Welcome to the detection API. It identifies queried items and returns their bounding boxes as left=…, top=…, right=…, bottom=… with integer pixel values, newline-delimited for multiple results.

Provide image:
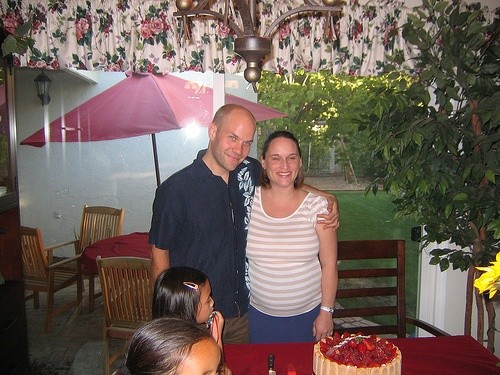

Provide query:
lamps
left=33, top=71, right=51, bottom=106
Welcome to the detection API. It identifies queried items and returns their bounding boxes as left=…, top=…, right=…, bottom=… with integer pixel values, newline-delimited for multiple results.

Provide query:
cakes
left=313, top=332, right=402, bottom=375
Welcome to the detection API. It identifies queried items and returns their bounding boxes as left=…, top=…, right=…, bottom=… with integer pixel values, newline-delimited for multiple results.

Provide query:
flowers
left=473, top=252, right=500, bottom=299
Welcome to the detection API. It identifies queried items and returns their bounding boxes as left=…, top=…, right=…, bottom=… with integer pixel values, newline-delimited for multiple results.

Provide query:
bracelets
left=320, top=305, right=333, bottom=313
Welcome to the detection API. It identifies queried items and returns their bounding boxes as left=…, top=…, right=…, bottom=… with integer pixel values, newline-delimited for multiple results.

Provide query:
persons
left=243, top=131, right=338, bottom=345
left=149, top=103, right=340, bottom=344
left=123, top=266, right=232, bottom=375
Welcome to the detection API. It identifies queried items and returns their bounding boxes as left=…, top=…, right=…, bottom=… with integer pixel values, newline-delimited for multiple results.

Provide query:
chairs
left=19, top=205, right=125, bottom=334
left=317, top=239, right=451, bottom=338
left=96, top=255, right=154, bottom=375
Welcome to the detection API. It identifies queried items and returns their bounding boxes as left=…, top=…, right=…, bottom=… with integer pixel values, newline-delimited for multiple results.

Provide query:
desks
left=79, top=232, right=152, bottom=313
left=222, top=336, right=500, bottom=375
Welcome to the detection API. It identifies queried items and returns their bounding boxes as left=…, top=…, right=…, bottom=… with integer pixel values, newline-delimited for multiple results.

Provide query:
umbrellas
left=20, top=63, right=289, bottom=186
